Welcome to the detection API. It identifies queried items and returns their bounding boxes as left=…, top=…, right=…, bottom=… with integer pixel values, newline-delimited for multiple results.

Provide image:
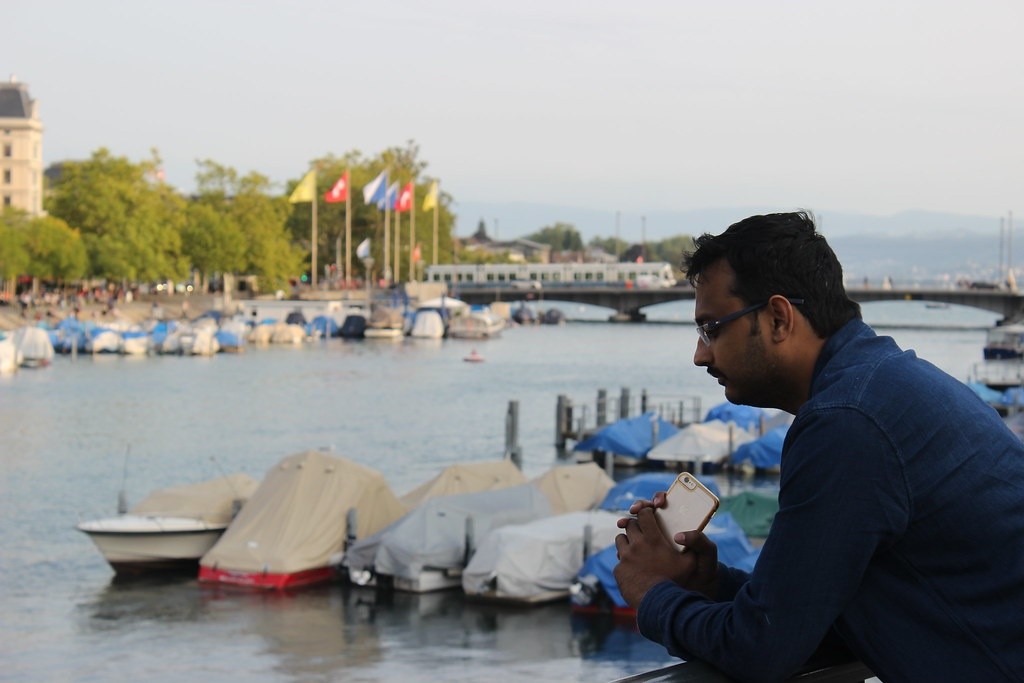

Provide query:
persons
left=615, top=212, right=1024, bottom=683
left=18, top=282, right=125, bottom=319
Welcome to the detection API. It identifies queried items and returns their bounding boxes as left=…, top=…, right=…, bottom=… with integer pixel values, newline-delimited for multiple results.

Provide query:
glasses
left=693, top=295, right=806, bottom=348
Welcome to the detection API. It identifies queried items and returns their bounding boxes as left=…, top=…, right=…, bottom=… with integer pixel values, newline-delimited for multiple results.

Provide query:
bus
left=427, top=262, right=679, bottom=288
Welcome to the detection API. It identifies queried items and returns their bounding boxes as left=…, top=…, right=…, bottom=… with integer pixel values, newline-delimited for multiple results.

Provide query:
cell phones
left=654, top=471, right=720, bottom=555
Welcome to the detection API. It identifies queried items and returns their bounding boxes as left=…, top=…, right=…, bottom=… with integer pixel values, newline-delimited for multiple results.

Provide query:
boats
left=339, top=485, right=552, bottom=596
left=1, top=301, right=568, bottom=376
left=458, top=503, right=630, bottom=604
left=198, top=449, right=403, bottom=590
left=73, top=471, right=257, bottom=577
left=731, top=428, right=786, bottom=477
left=568, top=523, right=755, bottom=632
left=647, top=420, right=760, bottom=476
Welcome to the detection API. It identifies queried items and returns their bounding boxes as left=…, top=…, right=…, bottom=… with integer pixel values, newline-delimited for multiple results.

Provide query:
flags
left=377, top=181, right=396, bottom=211
left=362, top=169, right=386, bottom=204
left=355, top=237, right=371, bottom=258
left=287, top=168, right=317, bottom=204
left=422, top=182, right=435, bottom=211
left=394, top=183, right=412, bottom=210
left=323, top=169, right=347, bottom=203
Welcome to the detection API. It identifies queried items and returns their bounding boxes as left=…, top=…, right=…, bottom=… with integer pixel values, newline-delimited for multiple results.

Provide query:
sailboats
left=575, top=409, right=682, bottom=467
